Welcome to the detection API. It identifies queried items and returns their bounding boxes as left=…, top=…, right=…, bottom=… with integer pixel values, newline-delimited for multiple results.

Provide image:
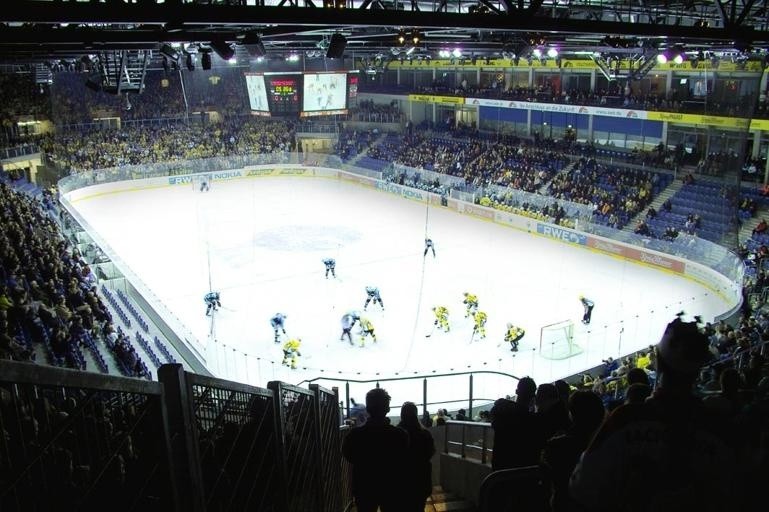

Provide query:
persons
left=204, top=291, right=222, bottom=316
left=433, top=305, right=450, bottom=332
left=341, top=312, right=376, bottom=347
left=424, top=238, right=435, bottom=256
left=324, top=258, right=336, bottom=278
left=0, top=226, right=117, bottom=361
left=2, top=323, right=769, bottom=511
left=271, top=313, right=286, bottom=342
left=736, top=219, right=768, bottom=328
left=364, top=286, right=384, bottom=311
left=463, top=293, right=487, bottom=338
left=579, top=295, right=593, bottom=323
left=1, top=50, right=769, bottom=227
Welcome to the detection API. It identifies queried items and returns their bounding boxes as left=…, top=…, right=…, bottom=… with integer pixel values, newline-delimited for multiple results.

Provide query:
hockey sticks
left=426, top=325, right=437, bottom=337
